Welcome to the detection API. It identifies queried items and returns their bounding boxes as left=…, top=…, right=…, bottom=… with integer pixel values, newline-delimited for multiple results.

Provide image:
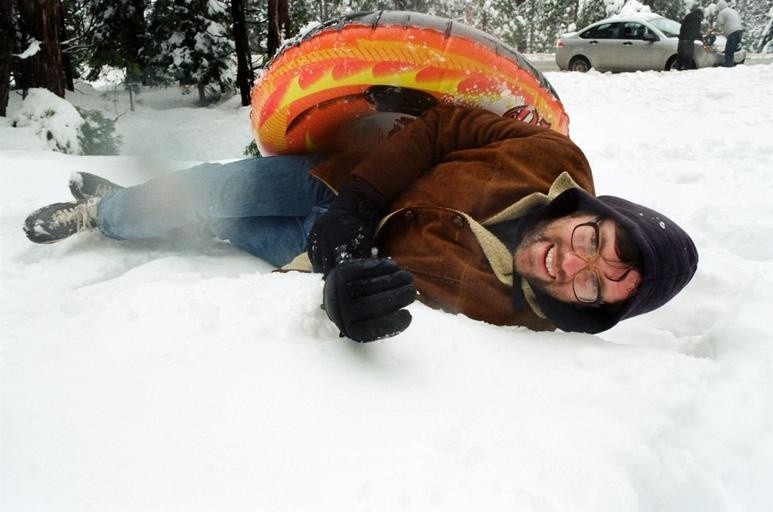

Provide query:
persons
left=709, top=0, right=743, bottom=67
left=22, top=102, right=699, bottom=341
left=673, top=7, right=704, bottom=71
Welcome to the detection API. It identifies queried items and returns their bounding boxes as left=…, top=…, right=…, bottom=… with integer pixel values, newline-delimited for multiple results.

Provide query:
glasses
left=571, top=215, right=604, bottom=309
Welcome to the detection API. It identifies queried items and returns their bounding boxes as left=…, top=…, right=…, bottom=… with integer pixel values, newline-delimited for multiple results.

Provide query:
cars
left=555, top=11, right=745, bottom=74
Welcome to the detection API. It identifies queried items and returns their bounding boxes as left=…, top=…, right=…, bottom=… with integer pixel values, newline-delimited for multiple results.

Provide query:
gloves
left=321, top=258, right=416, bottom=341
left=305, top=192, right=377, bottom=272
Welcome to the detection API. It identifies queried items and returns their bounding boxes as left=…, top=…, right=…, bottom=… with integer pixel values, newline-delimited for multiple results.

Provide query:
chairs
left=636, top=25, right=645, bottom=39
left=624, top=25, right=636, bottom=39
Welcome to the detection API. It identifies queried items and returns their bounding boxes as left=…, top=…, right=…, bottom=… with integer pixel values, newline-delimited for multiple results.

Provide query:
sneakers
left=70, top=172, right=124, bottom=201
left=24, top=201, right=97, bottom=244
